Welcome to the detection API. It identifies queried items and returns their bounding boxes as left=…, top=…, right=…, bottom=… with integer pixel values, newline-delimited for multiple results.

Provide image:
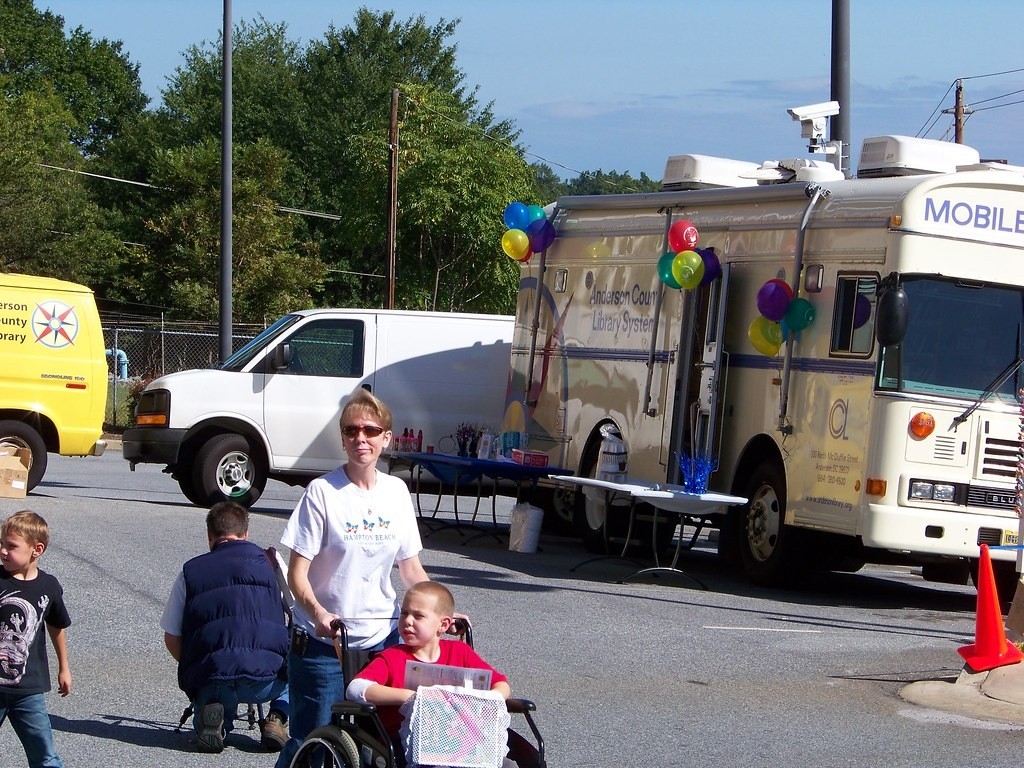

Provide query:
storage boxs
left=510, top=449, right=548, bottom=469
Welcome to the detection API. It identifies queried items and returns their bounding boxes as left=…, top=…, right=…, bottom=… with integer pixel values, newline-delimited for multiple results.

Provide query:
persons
left=0, top=509, right=72, bottom=768
left=343, top=582, right=531, bottom=768
left=275, top=388, right=471, bottom=768
left=160, top=502, right=295, bottom=750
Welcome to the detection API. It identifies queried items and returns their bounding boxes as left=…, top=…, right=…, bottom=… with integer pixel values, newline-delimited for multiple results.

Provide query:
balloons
left=527, top=205, right=546, bottom=223
left=748, top=279, right=814, bottom=357
left=580, top=242, right=613, bottom=270
left=656, top=251, right=684, bottom=289
left=699, top=249, right=720, bottom=287
left=504, top=202, right=530, bottom=231
left=672, top=251, right=705, bottom=290
left=516, top=247, right=532, bottom=262
left=668, top=219, right=699, bottom=253
left=528, top=219, right=556, bottom=254
left=853, top=293, right=872, bottom=331
left=502, top=229, right=529, bottom=260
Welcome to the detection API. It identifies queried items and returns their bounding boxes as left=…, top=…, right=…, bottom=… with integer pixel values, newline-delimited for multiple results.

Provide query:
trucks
left=0, top=272, right=108, bottom=493
left=500, top=101, right=1024, bottom=584
left=122, top=309, right=516, bottom=509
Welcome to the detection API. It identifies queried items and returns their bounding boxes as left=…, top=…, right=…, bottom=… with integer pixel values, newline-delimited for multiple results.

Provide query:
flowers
left=455, top=421, right=486, bottom=440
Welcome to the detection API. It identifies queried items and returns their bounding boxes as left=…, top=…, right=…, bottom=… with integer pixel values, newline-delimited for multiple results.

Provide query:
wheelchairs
left=289, top=618, right=548, bottom=768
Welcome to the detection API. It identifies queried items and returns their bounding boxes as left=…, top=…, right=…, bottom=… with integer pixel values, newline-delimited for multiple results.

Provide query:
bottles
left=400, top=428, right=424, bottom=452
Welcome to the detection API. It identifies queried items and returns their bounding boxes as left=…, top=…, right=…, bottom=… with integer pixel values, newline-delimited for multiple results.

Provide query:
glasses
left=342, top=425, right=385, bottom=436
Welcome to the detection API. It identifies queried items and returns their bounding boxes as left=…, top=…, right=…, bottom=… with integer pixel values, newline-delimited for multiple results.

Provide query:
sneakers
left=196, top=699, right=224, bottom=753
left=260, top=715, right=291, bottom=749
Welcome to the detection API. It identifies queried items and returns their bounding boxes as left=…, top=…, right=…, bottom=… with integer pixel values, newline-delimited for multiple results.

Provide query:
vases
left=457, top=438, right=478, bottom=459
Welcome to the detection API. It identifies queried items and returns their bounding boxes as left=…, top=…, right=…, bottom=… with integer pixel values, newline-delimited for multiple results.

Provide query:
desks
left=378, top=449, right=575, bottom=548
left=547, top=474, right=750, bottom=591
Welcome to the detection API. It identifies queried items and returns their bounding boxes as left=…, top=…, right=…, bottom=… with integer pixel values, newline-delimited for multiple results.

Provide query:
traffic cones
left=957, top=544, right=1023, bottom=672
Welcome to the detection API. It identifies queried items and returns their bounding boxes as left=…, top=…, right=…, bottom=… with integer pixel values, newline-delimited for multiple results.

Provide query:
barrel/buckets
left=509, top=508, right=544, bottom=553
